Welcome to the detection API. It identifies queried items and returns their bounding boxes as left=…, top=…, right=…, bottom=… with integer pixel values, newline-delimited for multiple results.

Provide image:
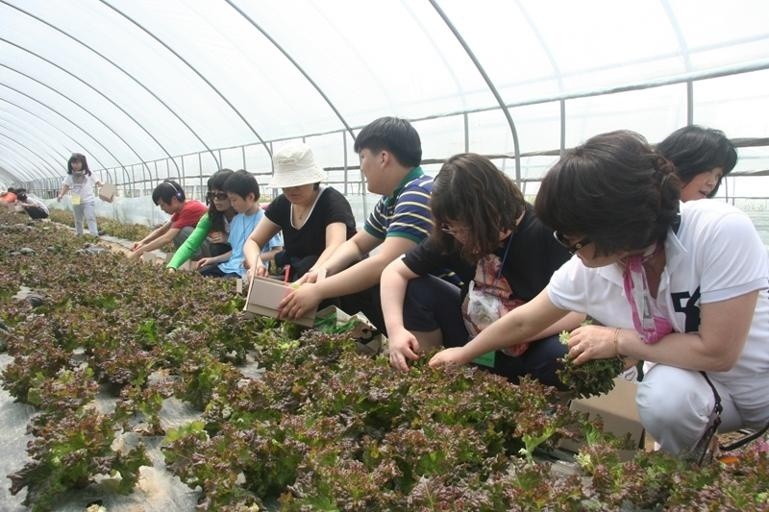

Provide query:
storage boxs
left=99, top=183, right=115, bottom=203
left=565, top=377, right=655, bottom=453
left=243, top=254, right=328, bottom=329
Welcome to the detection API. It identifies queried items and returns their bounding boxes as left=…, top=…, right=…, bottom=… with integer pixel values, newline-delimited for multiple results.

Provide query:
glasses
left=206, top=191, right=228, bottom=200
left=551, top=227, right=593, bottom=256
left=438, top=222, right=475, bottom=237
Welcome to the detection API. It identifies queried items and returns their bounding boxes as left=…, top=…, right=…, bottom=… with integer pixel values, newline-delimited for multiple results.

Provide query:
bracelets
left=613, top=326, right=622, bottom=355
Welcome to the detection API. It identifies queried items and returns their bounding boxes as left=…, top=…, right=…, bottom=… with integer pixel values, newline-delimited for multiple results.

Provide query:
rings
left=574, top=345, right=579, bottom=354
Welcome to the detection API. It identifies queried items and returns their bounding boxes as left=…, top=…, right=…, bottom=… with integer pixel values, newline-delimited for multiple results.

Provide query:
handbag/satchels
left=70, top=194, right=82, bottom=206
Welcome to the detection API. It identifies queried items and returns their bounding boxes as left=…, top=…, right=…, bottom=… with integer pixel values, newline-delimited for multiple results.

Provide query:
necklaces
left=295, top=202, right=310, bottom=220
left=242, top=208, right=259, bottom=242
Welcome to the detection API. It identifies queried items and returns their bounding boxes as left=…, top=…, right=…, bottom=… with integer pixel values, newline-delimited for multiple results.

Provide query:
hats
left=265, top=142, right=329, bottom=189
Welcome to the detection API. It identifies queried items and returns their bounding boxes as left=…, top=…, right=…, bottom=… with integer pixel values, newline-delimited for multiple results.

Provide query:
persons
left=279, top=117, right=436, bottom=338
left=0, top=187, right=49, bottom=222
left=242, top=142, right=357, bottom=285
left=427, top=130, right=769, bottom=455
left=378, top=153, right=576, bottom=390
left=56, top=154, right=104, bottom=237
left=166, top=168, right=237, bottom=276
left=195, top=169, right=284, bottom=278
left=655, top=124, right=738, bottom=204
left=129, top=178, right=210, bottom=262
